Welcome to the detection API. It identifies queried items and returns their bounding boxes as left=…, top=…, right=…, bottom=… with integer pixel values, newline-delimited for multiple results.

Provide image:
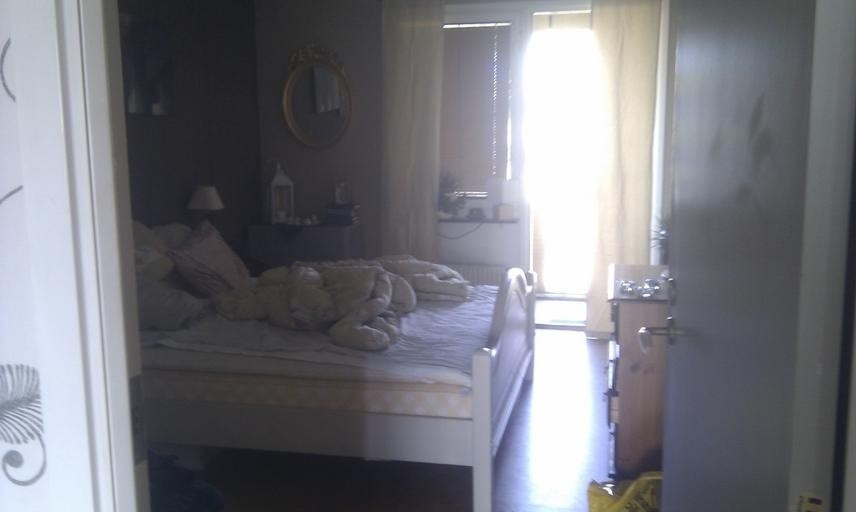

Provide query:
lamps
left=186, top=186, right=225, bottom=218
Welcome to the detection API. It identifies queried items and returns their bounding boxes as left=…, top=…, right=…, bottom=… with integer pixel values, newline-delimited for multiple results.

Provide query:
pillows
left=133, top=220, right=250, bottom=329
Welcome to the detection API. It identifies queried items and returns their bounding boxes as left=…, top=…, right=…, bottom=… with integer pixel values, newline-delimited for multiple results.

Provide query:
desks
left=248, top=222, right=364, bottom=268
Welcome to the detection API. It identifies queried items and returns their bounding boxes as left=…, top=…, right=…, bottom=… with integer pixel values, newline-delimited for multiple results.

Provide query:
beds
left=140, top=266, right=535, bottom=512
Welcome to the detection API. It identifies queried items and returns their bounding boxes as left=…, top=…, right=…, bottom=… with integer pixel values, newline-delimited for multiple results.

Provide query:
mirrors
left=282, top=43, right=353, bottom=150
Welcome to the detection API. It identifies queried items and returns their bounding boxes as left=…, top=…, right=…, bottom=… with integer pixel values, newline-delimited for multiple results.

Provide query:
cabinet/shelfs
left=606, top=265, right=668, bottom=479
